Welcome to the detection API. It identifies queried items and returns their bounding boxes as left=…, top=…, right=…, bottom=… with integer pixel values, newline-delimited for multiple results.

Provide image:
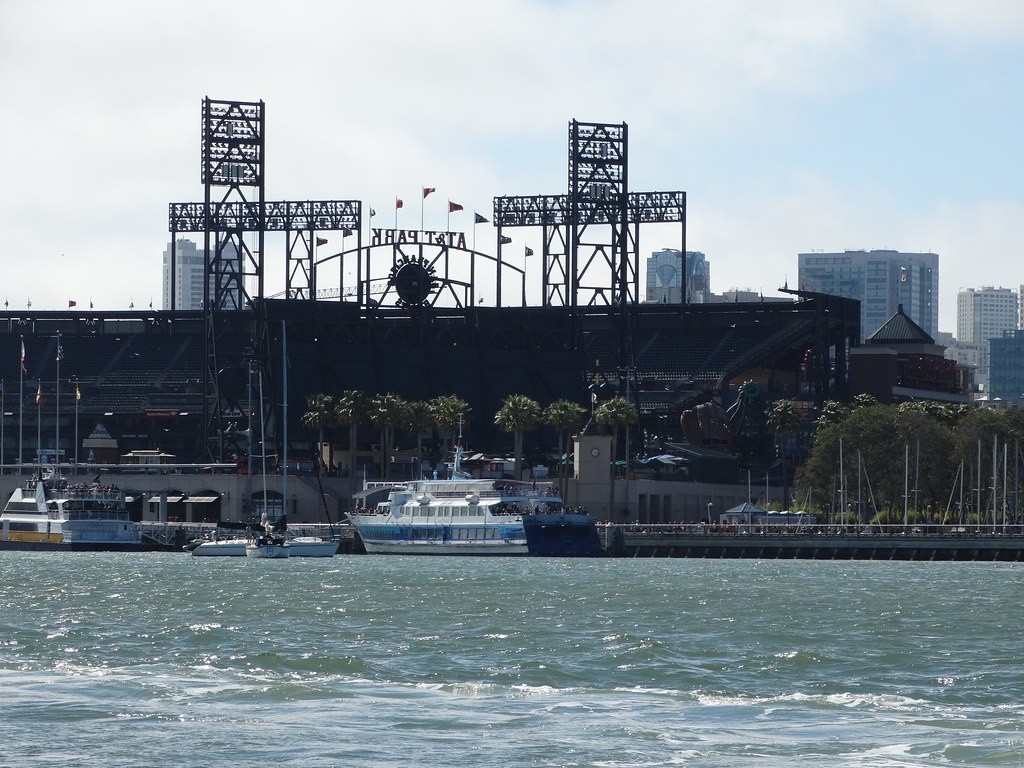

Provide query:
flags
left=58, top=345, right=65, bottom=360
left=424, top=188, right=435, bottom=198
left=525, top=247, right=533, bottom=256
left=450, top=202, right=463, bottom=212
left=5, top=300, right=8, bottom=307
left=397, top=199, right=402, bottom=208
left=317, top=237, right=328, bottom=246
left=500, top=235, right=511, bottom=244
left=21, top=340, right=25, bottom=363
left=591, top=393, right=598, bottom=403
left=69, top=301, right=76, bottom=307
left=344, top=228, right=352, bottom=237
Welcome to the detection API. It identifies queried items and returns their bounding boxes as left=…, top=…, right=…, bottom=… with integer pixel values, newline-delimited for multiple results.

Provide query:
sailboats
left=837, top=434, right=1024, bottom=534
left=182, top=320, right=341, bottom=559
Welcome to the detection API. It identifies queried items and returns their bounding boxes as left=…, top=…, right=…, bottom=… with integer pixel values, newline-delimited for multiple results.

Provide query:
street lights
left=707, top=502, right=713, bottom=525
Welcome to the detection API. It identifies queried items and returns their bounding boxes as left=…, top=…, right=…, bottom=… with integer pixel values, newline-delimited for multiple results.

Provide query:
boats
left=0, top=459, right=144, bottom=552
left=344, top=413, right=608, bottom=557
